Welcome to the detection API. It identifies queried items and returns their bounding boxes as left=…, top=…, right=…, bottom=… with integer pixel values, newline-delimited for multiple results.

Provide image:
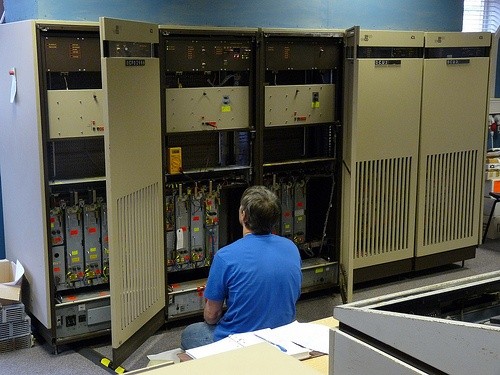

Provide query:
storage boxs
left=0, top=312, right=31, bottom=340
left=0, top=259, right=25, bottom=304
left=0, top=333, right=35, bottom=353
left=0, top=304, right=25, bottom=324
left=484, top=148, right=500, bottom=239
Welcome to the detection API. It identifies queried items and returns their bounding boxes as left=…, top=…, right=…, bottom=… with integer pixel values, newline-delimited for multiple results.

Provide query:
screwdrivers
left=255, top=335, right=287, bottom=352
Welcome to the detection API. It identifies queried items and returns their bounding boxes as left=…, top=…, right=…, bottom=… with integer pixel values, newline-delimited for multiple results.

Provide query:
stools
left=482, top=192, right=500, bottom=244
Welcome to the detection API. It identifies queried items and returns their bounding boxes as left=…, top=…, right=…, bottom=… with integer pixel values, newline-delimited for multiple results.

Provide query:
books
left=185, top=327, right=311, bottom=360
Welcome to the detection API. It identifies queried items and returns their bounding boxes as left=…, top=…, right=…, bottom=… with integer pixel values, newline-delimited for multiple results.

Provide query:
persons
left=181, top=185, right=302, bottom=352
